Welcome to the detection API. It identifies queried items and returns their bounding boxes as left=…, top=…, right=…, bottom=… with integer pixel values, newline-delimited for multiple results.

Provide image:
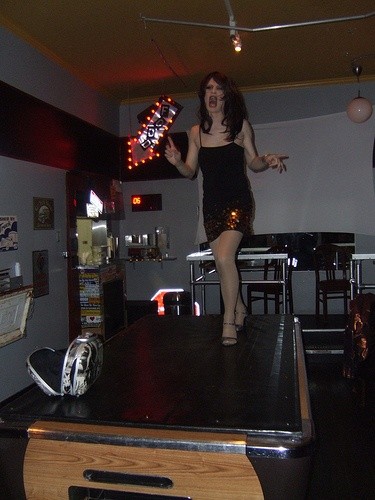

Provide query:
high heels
left=221, top=319, right=238, bottom=347
left=236, top=305, right=248, bottom=331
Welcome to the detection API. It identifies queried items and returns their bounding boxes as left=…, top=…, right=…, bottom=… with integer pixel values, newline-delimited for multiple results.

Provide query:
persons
left=162, top=69, right=289, bottom=347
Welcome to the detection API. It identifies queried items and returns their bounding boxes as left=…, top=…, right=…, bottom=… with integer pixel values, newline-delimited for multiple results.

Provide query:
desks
left=341, top=246, right=375, bottom=318
left=186, top=248, right=288, bottom=315
left=0, top=314, right=318, bottom=499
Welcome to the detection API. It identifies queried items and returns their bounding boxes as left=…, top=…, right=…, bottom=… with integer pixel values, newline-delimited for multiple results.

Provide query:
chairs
left=312, top=243, right=359, bottom=326
left=247, top=248, right=294, bottom=315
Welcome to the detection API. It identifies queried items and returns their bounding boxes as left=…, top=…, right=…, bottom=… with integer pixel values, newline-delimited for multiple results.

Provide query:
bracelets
left=261, top=153, right=272, bottom=167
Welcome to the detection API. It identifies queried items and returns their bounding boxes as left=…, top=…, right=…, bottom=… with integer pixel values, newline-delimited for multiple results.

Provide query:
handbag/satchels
left=25, top=332, right=108, bottom=399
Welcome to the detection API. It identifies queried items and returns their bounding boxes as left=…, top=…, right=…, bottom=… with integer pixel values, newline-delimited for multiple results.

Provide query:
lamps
left=228, top=21, right=242, bottom=53
left=346, top=63, right=372, bottom=123
